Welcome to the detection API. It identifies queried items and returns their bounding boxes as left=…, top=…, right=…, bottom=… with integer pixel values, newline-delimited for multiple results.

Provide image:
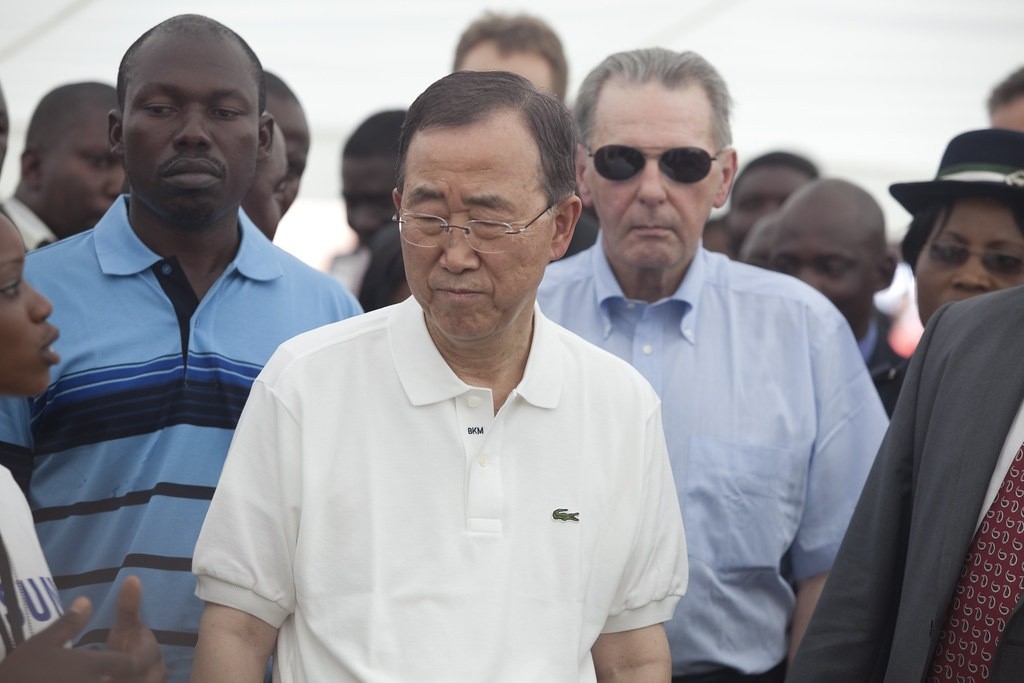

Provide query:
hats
left=888, top=129, right=1024, bottom=217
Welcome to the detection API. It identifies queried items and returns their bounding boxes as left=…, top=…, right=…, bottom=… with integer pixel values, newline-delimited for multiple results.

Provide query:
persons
left=190, top=70, right=689, bottom=683
left=0, top=13, right=412, bottom=683
left=452, top=13, right=1024, bottom=683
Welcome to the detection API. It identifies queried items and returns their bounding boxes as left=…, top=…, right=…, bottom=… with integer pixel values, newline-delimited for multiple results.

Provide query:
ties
left=925, top=441, right=1024, bottom=683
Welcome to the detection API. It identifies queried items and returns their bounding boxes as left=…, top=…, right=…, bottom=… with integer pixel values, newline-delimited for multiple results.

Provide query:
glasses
left=585, top=143, right=720, bottom=183
left=393, top=196, right=566, bottom=254
left=916, top=242, right=1024, bottom=276
